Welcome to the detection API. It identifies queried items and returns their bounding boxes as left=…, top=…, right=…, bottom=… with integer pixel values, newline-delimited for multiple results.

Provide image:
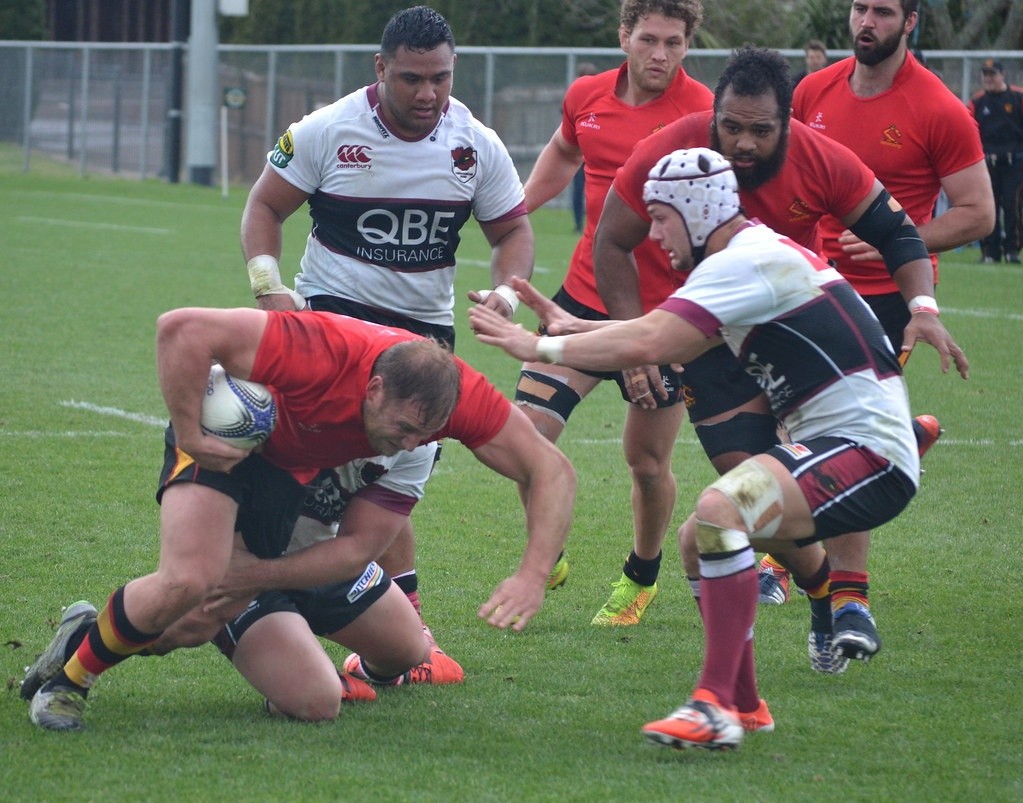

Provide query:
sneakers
left=28, top=675, right=88, bottom=734
left=912, top=412, right=944, bottom=474
left=736, top=700, right=776, bottom=734
left=19, top=601, right=100, bottom=703
left=344, top=646, right=464, bottom=690
left=831, top=598, right=881, bottom=665
left=492, top=555, right=570, bottom=626
left=808, top=625, right=851, bottom=674
left=757, top=557, right=789, bottom=605
left=640, top=685, right=744, bottom=753
left=340, top=671, right=378, bottom=703
left=592, top=570, right=659, bottom=627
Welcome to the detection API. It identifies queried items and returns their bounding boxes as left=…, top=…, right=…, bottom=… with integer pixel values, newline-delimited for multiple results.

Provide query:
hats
left=644, top=146, right=741, bottom=250
left=978, top=59, right=1004, bottom=77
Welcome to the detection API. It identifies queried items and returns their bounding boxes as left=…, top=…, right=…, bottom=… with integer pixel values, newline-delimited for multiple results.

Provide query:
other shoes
left=981, top=253, right=991, bottom=263
left=1003, top=252, right=1020, bottom=263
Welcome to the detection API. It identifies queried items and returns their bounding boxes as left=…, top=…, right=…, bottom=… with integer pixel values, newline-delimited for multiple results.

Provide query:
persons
left=593, top=48, right=972, bottom=675
left=513, top=0, right=713, bottom=624
left=17, top=307, right=576, bottom=726
left=962, top=59, right=1023, bottom=262
left=790, top=39, right=828, bottom=86
left=558, top=62, right=607, bottom=229
left=237, top=6, right=535, bottom=661
left=468, top=147, right=922, bottom=750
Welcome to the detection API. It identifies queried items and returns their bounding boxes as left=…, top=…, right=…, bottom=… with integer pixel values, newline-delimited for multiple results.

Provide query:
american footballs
left=200, top=362, right=278, bottom=449
